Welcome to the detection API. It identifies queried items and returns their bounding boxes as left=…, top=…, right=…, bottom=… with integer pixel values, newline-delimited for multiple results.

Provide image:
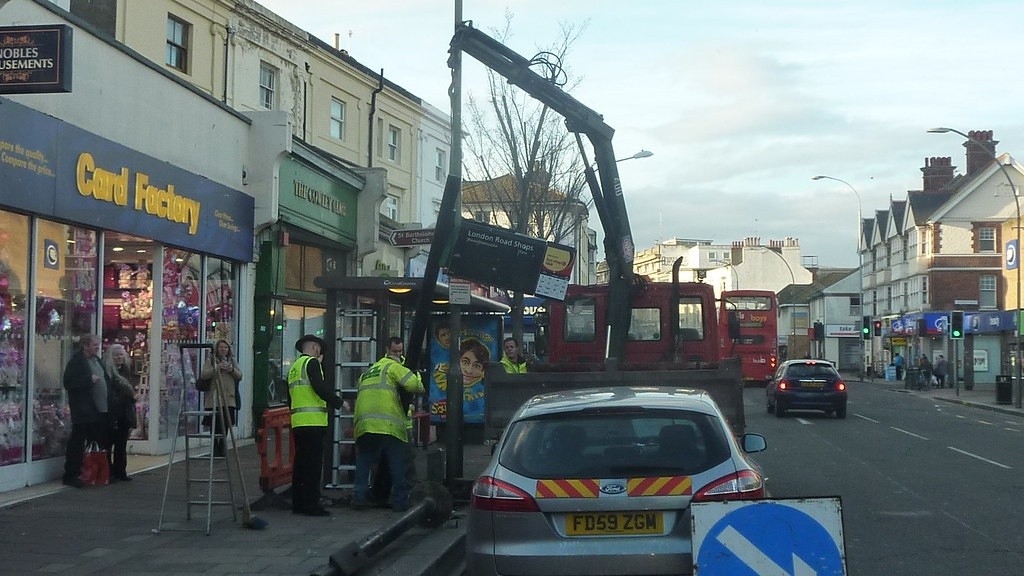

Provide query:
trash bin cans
left=993, top=374, right=1013, bottom=405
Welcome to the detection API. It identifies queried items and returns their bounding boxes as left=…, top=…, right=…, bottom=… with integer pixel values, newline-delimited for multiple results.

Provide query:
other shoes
left=392, top=501, right=412, bottom=511
left=114, top=471, right=132, bottom=481
left=304, top=507, right=331, bottom=516
left=220, top=441, right=228, bottom=455
left=63, top=473, right=81, bottom=486
left=214, top=440, right=219, bottom=456
left=292, top=507, right=303, bottom=516
left=351, top=496, right=374, bottom=507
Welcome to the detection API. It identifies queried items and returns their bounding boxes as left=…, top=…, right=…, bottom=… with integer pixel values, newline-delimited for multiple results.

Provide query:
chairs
left=534, top=423, right=704, bottom=477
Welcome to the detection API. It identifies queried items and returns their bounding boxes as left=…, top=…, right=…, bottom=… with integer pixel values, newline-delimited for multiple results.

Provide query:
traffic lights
left=874, top=320, right=882, bottom=337
left=950, top=310, right=965, bottom=341
left=862, top=315, right=871, bottom=340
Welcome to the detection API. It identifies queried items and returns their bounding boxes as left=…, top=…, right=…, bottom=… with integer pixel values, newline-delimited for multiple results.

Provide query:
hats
left=295, top=334, right=325, bottom=354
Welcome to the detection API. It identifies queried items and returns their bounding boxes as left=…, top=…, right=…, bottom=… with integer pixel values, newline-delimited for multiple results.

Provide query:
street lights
left=709, top=258, right=740, bottom=291
left=812, top=174, right=866, bottom=382
left=573, top=148, right=654, bottom=286
left=926, top=127, right=1024, bottom=410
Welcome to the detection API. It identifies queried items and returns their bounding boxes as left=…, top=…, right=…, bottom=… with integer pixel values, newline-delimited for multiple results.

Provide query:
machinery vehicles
left=396, top=1, right=751, bottom=451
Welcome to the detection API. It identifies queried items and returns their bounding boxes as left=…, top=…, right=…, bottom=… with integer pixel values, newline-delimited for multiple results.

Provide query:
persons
left=895, top=352, right=947, bottom=389
left=500, top=338, right=528, bottom=373
left=63, top=334, right=114, bottom=488
left=354, top=355, right=427, bottom=512
left=287, top=334, right=350, bottom=517
left=200, top=339, right=243, bottom=457
left=369, top=337, right=418, bottom=485
left=269, top=361, right=286, bottom=403
left=101, top=344, right=138, bottom=481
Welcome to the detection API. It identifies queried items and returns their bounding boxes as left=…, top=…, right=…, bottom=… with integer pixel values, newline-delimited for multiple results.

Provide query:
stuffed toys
left=120, top=270, right=153, bottom=319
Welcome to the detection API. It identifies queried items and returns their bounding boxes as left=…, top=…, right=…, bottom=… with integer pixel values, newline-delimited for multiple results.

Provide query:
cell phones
left=222, top=357, right=227, bottom=364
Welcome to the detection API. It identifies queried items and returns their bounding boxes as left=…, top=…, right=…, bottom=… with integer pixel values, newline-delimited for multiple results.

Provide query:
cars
left=464, top=384, right=770, bottom=576
left=765, top=359, right=848, bottom=419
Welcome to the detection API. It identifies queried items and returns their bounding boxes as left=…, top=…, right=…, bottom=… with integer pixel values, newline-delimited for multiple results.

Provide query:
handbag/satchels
left=196, top=377, right=210, bottom=392
left=81, top=441, right=109, bottom=486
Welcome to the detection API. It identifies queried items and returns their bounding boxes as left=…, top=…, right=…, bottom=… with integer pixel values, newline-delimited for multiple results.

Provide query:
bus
left=716, top=288, right=780, bottom=384
